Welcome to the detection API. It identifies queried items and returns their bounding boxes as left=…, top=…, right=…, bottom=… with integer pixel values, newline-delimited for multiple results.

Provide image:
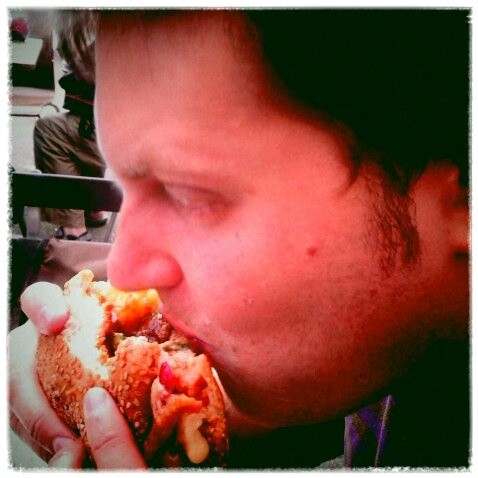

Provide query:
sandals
left=52, top=226, right=92, bottom=241
left=85, top=210, right=108, bottom=227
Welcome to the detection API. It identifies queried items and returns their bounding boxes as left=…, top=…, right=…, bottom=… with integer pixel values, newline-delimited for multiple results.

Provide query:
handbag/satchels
left=24, top=239, right=113, bottom=291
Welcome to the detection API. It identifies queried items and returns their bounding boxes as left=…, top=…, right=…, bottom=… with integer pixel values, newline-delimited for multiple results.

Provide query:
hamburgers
left=35, top=269, right=232, bottom=471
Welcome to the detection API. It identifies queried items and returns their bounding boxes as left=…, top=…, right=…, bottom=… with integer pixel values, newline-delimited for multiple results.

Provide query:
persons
left=32, top=38, right=108, bottom=240
left=9, top=4, right=469, bottom=468
